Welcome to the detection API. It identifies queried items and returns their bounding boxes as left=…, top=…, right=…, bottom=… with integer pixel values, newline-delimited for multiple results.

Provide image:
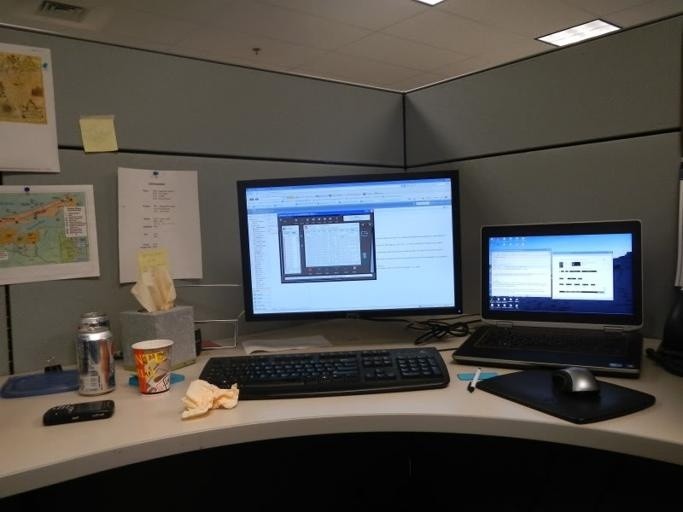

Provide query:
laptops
left=452, top=218, right=644, bottom=379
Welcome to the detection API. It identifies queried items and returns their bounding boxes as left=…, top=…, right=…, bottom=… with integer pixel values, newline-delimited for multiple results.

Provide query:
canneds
left=79, top=311, right=110, bottom=328
left=76, top=326, right=116, bottom=396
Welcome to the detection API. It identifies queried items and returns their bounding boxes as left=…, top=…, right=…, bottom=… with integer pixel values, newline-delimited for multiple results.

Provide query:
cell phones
left=43, top=400, right=114, bottom=426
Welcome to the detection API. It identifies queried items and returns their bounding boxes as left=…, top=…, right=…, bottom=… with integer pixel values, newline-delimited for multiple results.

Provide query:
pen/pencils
left=468, top=367, right=481, bottom=393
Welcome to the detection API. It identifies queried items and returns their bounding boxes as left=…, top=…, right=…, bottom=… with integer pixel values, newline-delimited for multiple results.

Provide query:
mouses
left=552, top=366, right=600, bottom=400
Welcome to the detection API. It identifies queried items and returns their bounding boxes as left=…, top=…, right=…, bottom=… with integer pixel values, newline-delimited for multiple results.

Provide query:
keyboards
left=199, top=347, right=451, bottom=401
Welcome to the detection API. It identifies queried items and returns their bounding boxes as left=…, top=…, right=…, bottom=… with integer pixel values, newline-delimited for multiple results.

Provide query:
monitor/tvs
left=236, top=169, right=464, bottom=348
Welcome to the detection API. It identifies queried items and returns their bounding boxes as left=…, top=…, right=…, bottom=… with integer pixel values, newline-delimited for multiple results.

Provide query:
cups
left=131, top=339, right=175, bottom=394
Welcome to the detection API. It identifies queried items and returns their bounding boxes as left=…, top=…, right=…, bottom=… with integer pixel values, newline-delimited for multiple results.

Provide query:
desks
left=0, top=314, right=683, bottom=512
